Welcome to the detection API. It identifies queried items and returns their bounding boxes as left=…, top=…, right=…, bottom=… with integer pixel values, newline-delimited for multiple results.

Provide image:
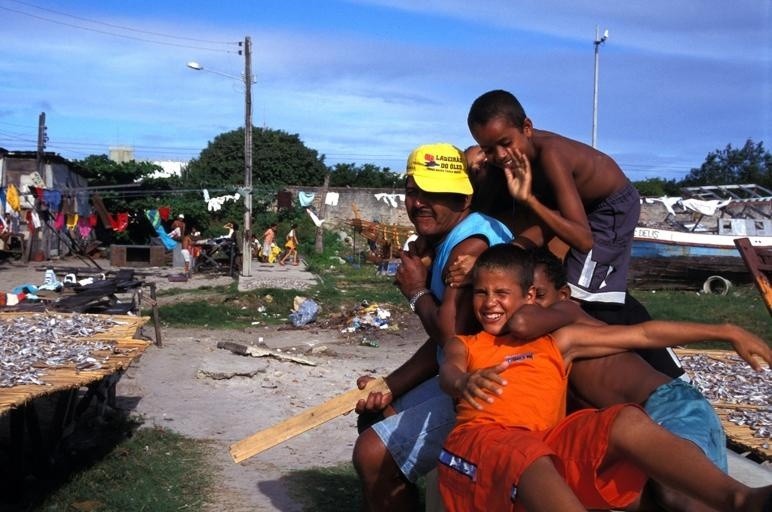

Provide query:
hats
left=406, top=143, right=475, bottom=197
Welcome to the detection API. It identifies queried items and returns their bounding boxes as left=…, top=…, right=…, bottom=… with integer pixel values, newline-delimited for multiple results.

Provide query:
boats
left=630, top=225, right=772, bottom=276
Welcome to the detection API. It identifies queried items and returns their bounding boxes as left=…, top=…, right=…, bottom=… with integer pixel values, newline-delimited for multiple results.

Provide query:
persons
left=375, top=240, right=391, bottom=276
left=352, top=143, right=523, bottom=512
left=403, top=230, right=419, bottom=252
left=507, top=247, right=728, bottom=512
left=171, top=213, right=282, bottom=277
left=280, top=223, right=301, bottom=266
left=444, top=89, right=693, bottom=385
left=437, top=243, right=771, bottom=512
left=368, top=219, right=380, bottom=254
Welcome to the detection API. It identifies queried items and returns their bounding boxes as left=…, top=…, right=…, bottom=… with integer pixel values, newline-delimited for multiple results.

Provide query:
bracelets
left=410, top=289, right=429, bottom=313
